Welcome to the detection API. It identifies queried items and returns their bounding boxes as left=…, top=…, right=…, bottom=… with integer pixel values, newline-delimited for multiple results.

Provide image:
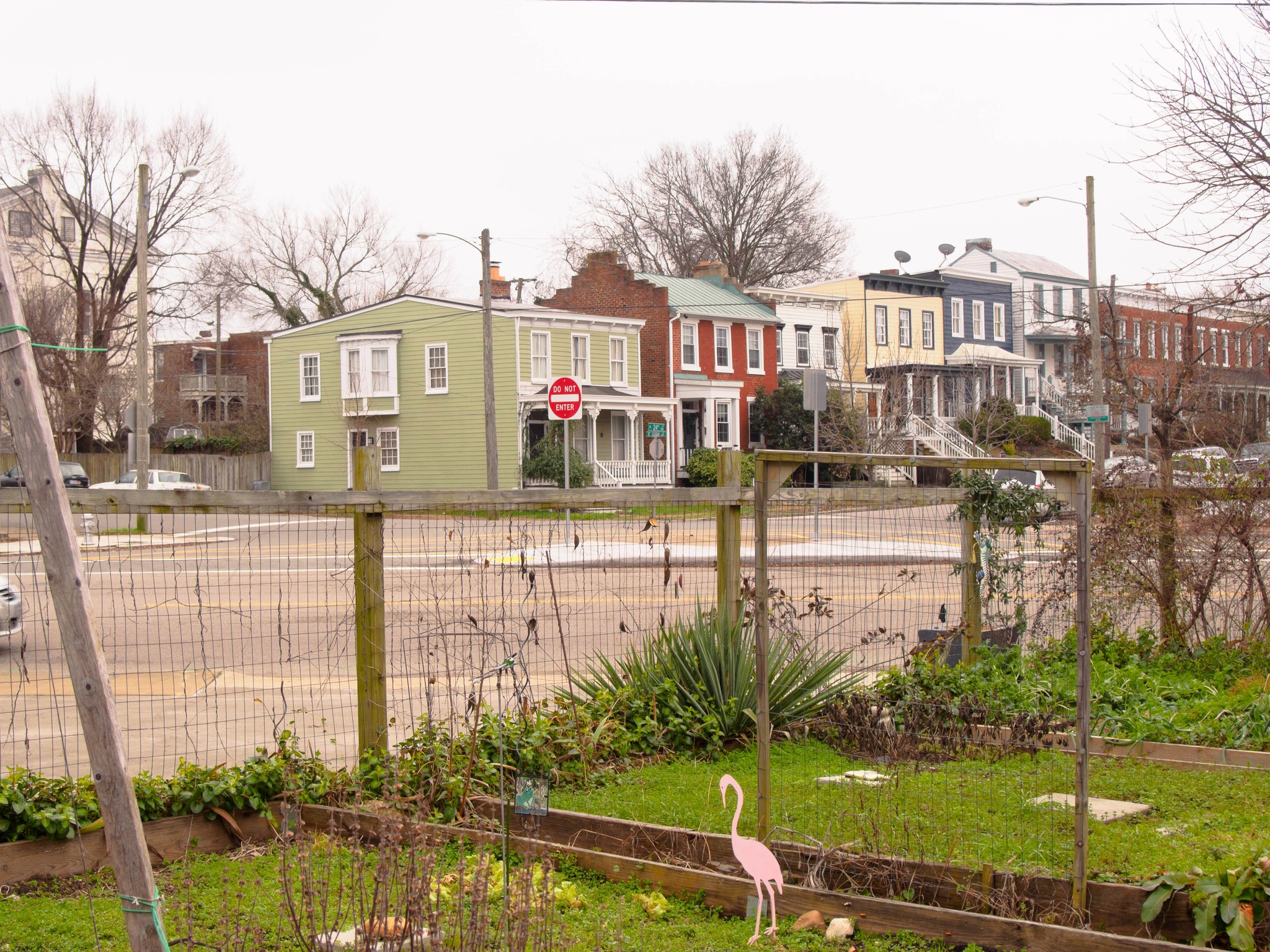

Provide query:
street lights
left=1016, top=174, right=1107, bottom=512
left=135, top=162, right=201, bottom=535
left=416, top=227, right=500, bottom=493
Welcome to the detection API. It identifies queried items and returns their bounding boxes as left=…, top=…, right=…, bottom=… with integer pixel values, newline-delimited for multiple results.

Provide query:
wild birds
left=719, top=774, right=784, bottom=945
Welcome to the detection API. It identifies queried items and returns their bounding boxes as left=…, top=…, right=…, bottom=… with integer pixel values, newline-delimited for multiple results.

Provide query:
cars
left=1172, top=441, right=1270, bottom=487
left=0, top=459, right=91, bottom=489
left=89, top=467, right=212, bottom=491
left=985, top=469, right=1065, bottom=526
left=1088, top=454, right=1162, bottom=491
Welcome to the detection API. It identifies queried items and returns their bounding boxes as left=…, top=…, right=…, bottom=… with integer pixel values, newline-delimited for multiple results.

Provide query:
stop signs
left=547, top=375, right=582, bottom=420
